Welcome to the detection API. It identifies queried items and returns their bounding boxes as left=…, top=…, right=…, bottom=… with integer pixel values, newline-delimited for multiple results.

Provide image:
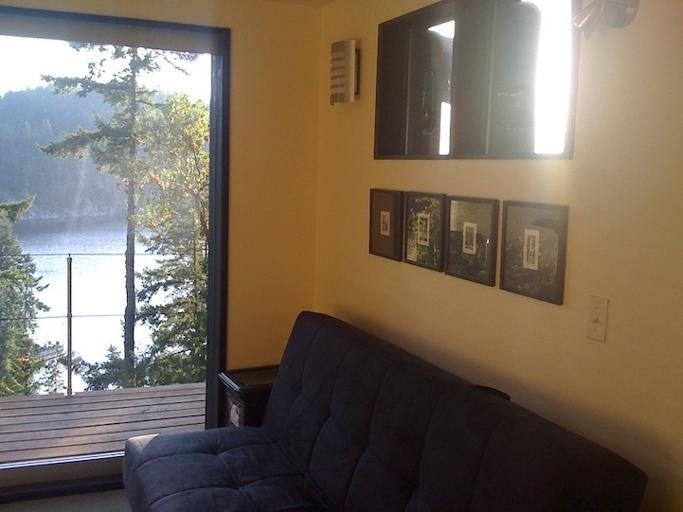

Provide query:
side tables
left=217, top=364, right=276, bottom=429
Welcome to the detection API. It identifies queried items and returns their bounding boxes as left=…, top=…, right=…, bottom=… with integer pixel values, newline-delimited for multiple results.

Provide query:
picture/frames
left=368, top=188, right=403, bottom=262
left=402, top=190, right=445, bottom=272
left=499, top=200, right=569, bottom=305
left=444, top=195, right=500, bottom=287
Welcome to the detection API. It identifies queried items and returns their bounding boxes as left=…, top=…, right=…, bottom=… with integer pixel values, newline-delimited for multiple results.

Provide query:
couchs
left=122, top=311, right=648, bottom=512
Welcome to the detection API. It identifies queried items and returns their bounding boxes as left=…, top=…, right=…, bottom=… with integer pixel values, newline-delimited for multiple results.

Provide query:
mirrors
left=373, top=0, right=579, bottom=160
